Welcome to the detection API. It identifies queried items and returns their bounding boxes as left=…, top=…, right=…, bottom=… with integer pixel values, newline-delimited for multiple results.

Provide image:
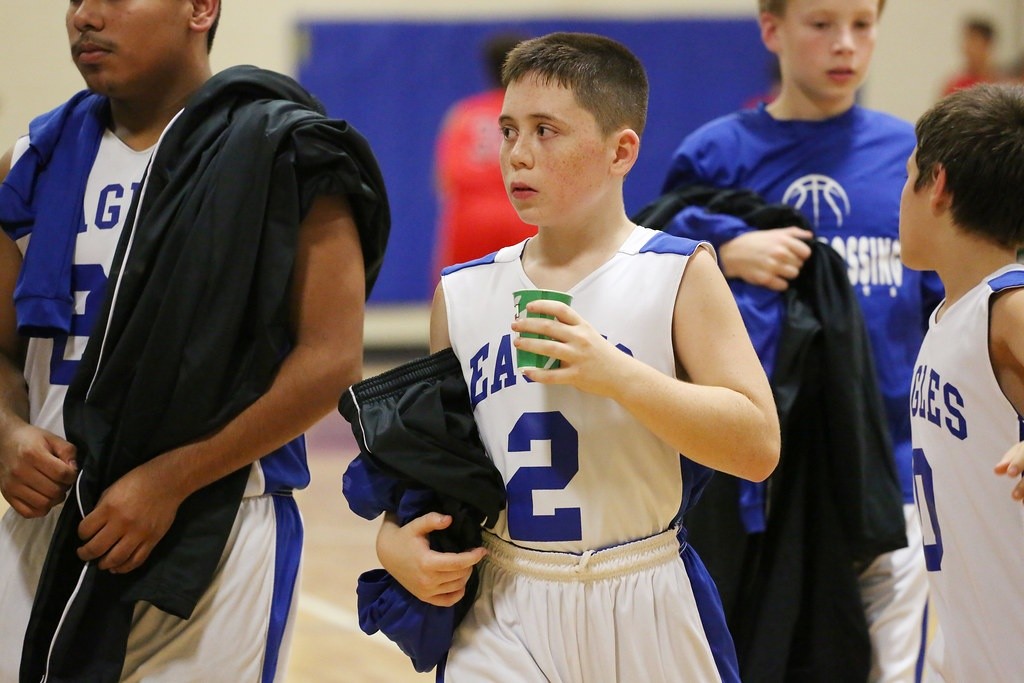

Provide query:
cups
left=512, top=289, right=573, bottom=374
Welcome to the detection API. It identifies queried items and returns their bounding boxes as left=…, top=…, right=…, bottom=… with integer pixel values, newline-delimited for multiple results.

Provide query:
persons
left=369, top=31, right=780, bottom=683
left=655, top=1, right=943, bottom=682
left=894, top=85, right=1023, bottom=682
left=1, top=1, right=366, bottom=682
